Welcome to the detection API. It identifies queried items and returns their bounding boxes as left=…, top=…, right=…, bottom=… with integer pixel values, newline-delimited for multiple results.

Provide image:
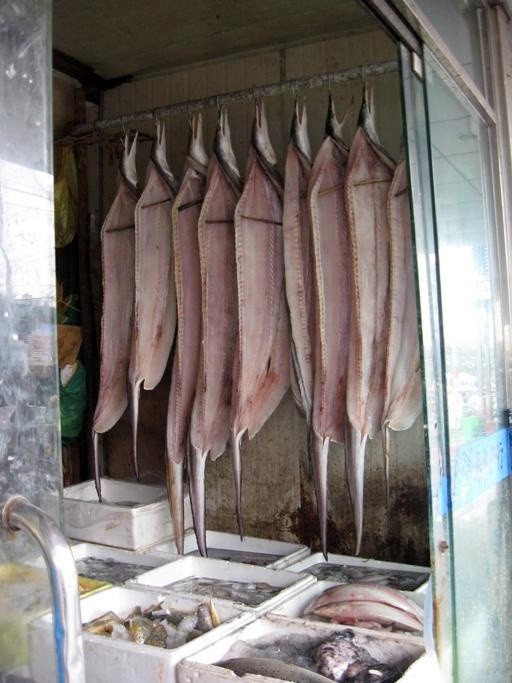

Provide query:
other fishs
left=296, top=563, right=431, bottom=591
left=89, top=80, right=425, bottom=562
left=80, top=591, right=222, bottom=649
left=160, top=574, right=285, bottom=608
left=312, top=600, right=423, bottom=632
left=303, top=582, right=424, bottom=625
left=210, top=656, right=339, bottom=683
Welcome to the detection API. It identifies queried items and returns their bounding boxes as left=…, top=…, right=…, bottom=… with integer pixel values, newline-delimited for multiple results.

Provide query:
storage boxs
left=0, top=562, right=110, bottom=683
left=265, top=581, right=430, bottom=645
left=27, top=584, right=257, bottom=683
left=39, top=476, right=195, bottom=551
left=144, top=528, right=312, bottom=570
left=126, top=555, right=319, bottom=614
left=26, top=542, right=181, bottom=586
left=176, top=612, right=434, bottom=683
left=284, top=550, right=431, bottom=593
